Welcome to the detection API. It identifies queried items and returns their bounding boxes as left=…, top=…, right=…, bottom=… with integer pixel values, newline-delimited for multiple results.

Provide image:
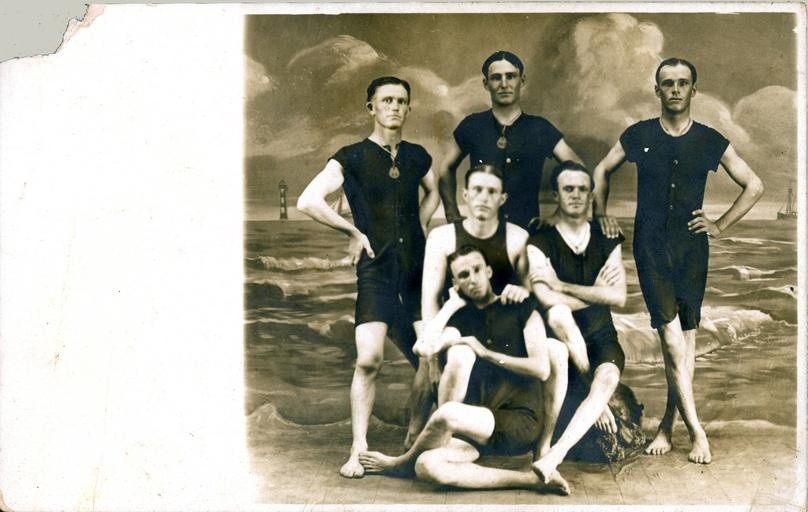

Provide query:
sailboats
left=777, top=189, right=797, bottom=219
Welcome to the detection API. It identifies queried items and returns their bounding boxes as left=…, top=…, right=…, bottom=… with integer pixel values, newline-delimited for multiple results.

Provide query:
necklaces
left=496, top=113, right=521, bottom=149
left=378, top=143, right=400, bottom=179
left=558, top=224, right=587, bottom=249
left=661, top=117, right=691, bottom=135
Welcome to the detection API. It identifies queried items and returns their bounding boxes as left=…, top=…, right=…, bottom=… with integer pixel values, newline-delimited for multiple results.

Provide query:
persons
left=525, top=160, right=627, bottom=486
left=358, top=244, right=570, bottom=495
left=438, top=51, right=585, bottom=235
left=592, top=57, right=764, bottom=463
left=421, top=163, right=569, bottom=462
left=296, top=76, right=441, bottom=478
